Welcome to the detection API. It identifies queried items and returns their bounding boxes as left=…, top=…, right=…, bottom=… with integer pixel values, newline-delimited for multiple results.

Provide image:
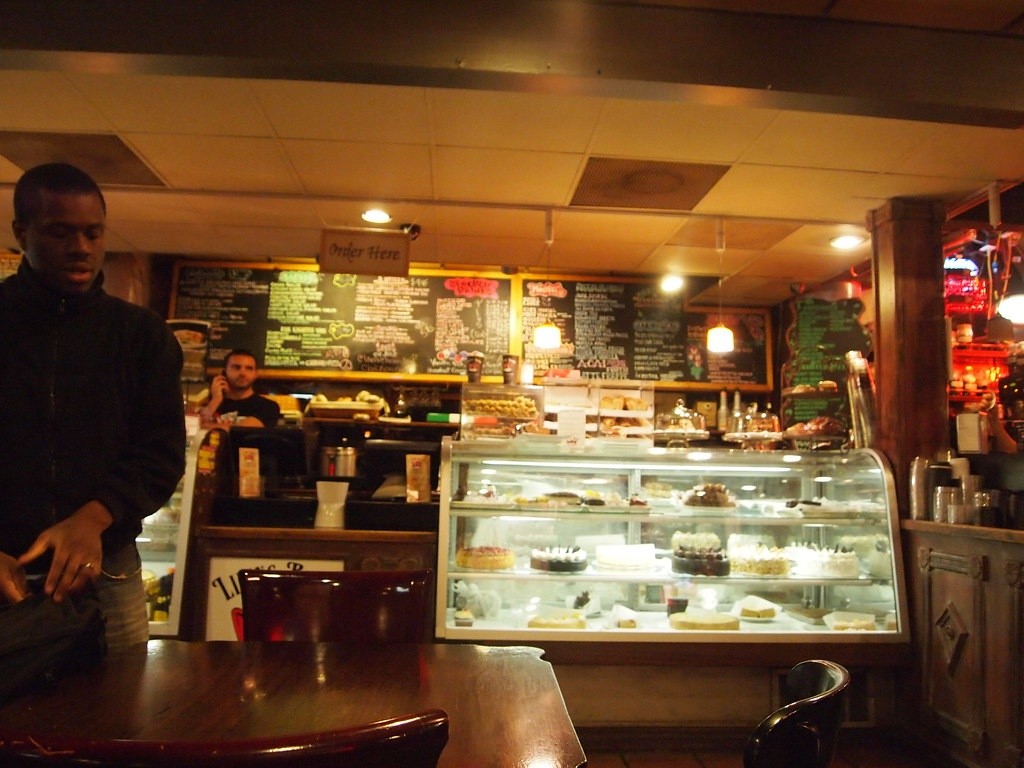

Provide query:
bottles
left=394, top=384, right=406, bottom=414
left=910, top=457, right=929, bottom=520
left=520, top=359, right=534, bottom=384
left=950, top=363, right=1001, bottom=389
left=717, top=390, right=730, bottom=431
left=957, top=324, right=972, bottom=343
left=731, top=392, right=741, bottom=415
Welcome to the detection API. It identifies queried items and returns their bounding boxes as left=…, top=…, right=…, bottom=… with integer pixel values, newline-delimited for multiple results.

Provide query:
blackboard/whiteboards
left=168, top=261, right=775, bottom=392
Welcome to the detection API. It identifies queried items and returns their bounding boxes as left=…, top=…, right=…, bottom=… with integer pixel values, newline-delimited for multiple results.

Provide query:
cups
left=934, top=486, right=962, bottom=523
left=502, top=354, right=519, bottom=384
left=947, top=490, right=983, bottom=526
left=316, top=481, right=349, bottom=503
left=950, top=458, right=969, bottom=478
left=927, top=463, right=953, bottom=522
left=315, top=503, right=346, bottom=527
left=845, top=350, right=875, bottom=450
left=961, top=474, right=986, bottom=490
left=466, top=352, right=484, bottom=383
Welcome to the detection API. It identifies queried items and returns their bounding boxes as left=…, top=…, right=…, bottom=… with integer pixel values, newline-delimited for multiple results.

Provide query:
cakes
left=451, top=484, right=879, bottom=633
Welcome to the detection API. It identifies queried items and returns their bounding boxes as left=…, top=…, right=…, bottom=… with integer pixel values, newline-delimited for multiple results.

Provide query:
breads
left=585, top=395, right=644, bottom=440
left=793, top=380, right=838, bottom=392
left=668, top=418, right=695, bottom=431
left=786, top=417, right=844, bottom=432
left=465, top=397, right=538, bottom=440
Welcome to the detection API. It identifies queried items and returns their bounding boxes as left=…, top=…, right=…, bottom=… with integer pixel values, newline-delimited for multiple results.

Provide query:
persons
left=197, top=349, right=280, bottom=431
left=980, top=396, right=1024, bottom=454
left=0, top=163, right=187, bottom=644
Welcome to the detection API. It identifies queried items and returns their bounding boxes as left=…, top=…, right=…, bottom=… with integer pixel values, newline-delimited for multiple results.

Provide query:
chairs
left=1, top=696, right=447, bottom=768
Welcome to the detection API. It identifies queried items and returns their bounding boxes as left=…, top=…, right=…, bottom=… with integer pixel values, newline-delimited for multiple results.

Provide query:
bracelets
left=992, top=426, right=1003, bottom=434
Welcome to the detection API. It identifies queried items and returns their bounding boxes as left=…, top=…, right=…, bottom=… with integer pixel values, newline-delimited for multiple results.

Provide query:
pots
left=319, top=447, right=356, bottom=478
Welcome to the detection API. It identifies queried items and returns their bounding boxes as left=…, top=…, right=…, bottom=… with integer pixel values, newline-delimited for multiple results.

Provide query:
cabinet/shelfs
left=898, top=518, right=1024, bottom=768
left=207, top=366, right=465, bottom=506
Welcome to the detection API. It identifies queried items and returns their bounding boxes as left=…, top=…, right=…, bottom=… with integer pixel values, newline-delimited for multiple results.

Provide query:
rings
left=80, top=562, right=94, bottom=569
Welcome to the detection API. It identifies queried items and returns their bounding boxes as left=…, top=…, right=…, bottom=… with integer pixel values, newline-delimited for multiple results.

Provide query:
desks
left=0, top=634, right=589, bottom=768
